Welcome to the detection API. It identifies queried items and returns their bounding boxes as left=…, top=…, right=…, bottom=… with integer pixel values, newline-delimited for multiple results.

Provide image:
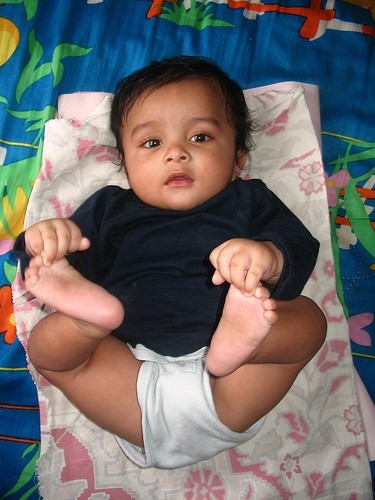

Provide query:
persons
left=13, top=57, right=328, bottom=469
